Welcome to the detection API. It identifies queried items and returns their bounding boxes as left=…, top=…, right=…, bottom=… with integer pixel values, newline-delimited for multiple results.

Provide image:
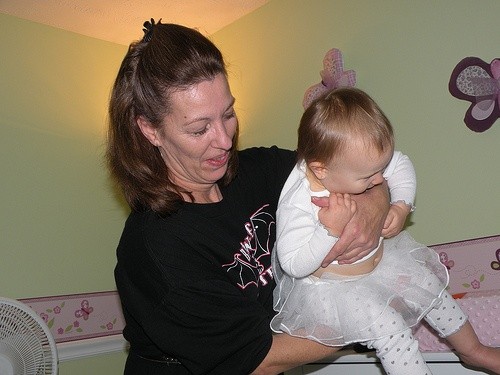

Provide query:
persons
left=106, top=17, right=391, bottom=375
left=269, top=86, right=500, bottom=375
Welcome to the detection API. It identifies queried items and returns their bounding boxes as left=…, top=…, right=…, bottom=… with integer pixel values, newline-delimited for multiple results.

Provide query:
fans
left=0, top=297, right=59, bottom=375
